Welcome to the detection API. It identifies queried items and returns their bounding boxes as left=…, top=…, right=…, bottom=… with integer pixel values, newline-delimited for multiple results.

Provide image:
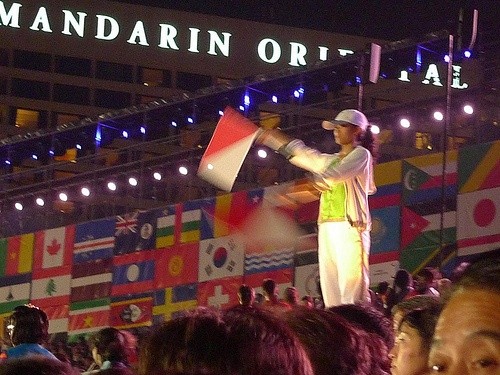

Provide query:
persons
left=254, top=109, right=379, bottom=309
left=0, top=259, right=500, bottom=375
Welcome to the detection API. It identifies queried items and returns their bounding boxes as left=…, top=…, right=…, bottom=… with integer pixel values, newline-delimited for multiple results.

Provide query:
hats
left=321, top=109, right=369, bottom=133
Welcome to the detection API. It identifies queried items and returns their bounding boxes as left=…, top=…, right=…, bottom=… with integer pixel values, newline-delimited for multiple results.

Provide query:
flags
left=196, top=106, right=261, bottom=193
